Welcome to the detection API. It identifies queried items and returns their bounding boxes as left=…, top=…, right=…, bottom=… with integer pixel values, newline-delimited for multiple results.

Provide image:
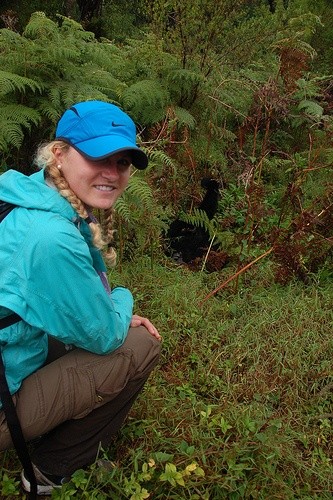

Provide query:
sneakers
left=21, top=459, right=116, bottom=496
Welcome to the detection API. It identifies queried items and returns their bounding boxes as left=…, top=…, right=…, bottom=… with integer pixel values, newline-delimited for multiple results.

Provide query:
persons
left=0, top=99, right=164, bottom=494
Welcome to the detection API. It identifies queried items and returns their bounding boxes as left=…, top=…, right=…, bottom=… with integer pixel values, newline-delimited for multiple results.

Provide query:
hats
left=55, top=100, right=148, bottom=170
